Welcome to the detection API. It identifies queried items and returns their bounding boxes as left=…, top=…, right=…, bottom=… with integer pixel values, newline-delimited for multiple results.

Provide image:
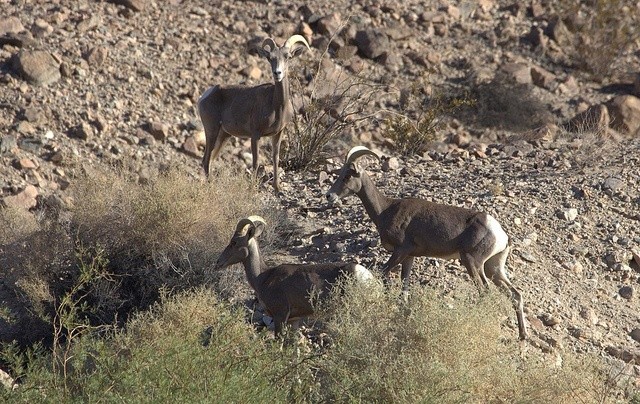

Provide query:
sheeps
left=197, top=34, right=315, bottom=198
left=216, top=215, right=383, bottom=360
left=326, top=145, right=530, bottom=342
